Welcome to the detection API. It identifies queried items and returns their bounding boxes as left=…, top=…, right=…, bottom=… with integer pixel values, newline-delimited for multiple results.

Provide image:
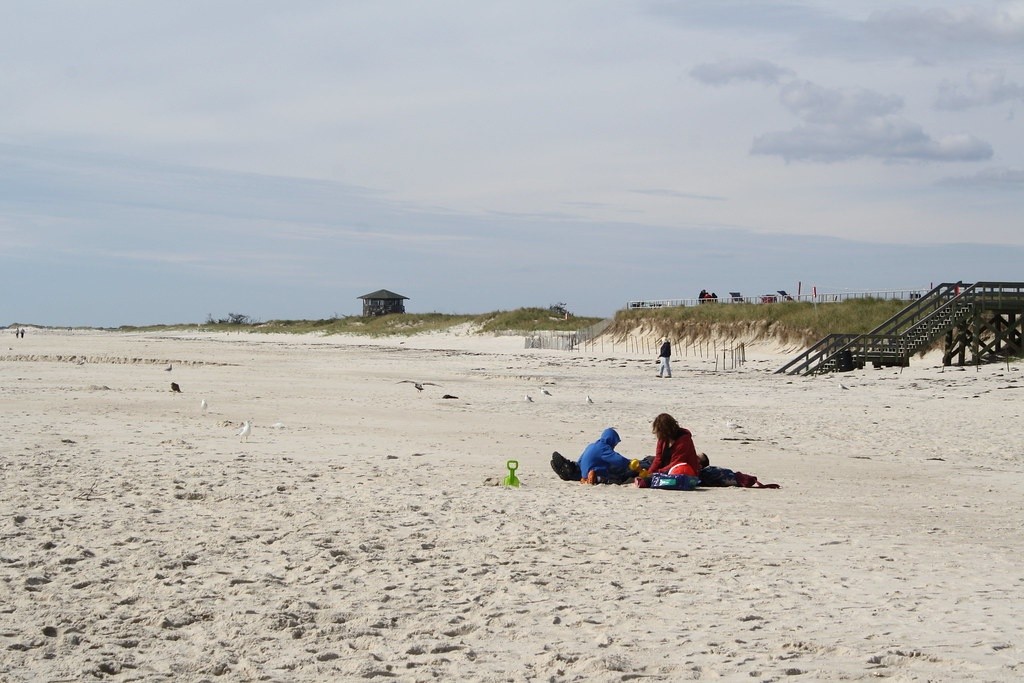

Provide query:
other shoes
left=587, top=470, right=607, bottom=485
left=656, top=376, right=662, bottom=378
left=635, top=477, right=646, bottom=488
left=665, top=376, right=671, bottom=378
left=550, top=452, right=578, bottom=480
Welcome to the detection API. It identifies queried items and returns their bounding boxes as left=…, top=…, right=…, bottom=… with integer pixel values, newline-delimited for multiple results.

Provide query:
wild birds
left=395, top=380, right=444, bottom=392
left=525, top=394, right=534, bottom=402
left=170, top=382, right=183, bottom=393
left=540, top=387, right=553, bottom=397
left=586, top=394, right=594, bottom=403
left=164, top=363, right=172, bottom=371
left=200, top=399, right=208, bottom=414
left=234, top=418, right=253, bottom=444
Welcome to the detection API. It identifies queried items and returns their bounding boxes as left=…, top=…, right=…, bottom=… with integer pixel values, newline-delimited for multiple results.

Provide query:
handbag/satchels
left=656, top=360, right=661, bottom=364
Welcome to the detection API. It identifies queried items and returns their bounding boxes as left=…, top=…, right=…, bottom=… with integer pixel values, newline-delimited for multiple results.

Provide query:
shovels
left=504, top=460, right=520, bottom=488
left=629, top=458, right=647, bottom=477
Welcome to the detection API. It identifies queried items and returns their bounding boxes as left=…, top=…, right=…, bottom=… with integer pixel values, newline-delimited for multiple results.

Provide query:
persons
left=588, top=413, right=699, bottom=487
left=549, top=449, right=709, bottom=481
left=698, top=289, right=717, bottom=304
left=655, top=336, right=671, bottom=378
left=577, top=428, right=628, bottom=482
